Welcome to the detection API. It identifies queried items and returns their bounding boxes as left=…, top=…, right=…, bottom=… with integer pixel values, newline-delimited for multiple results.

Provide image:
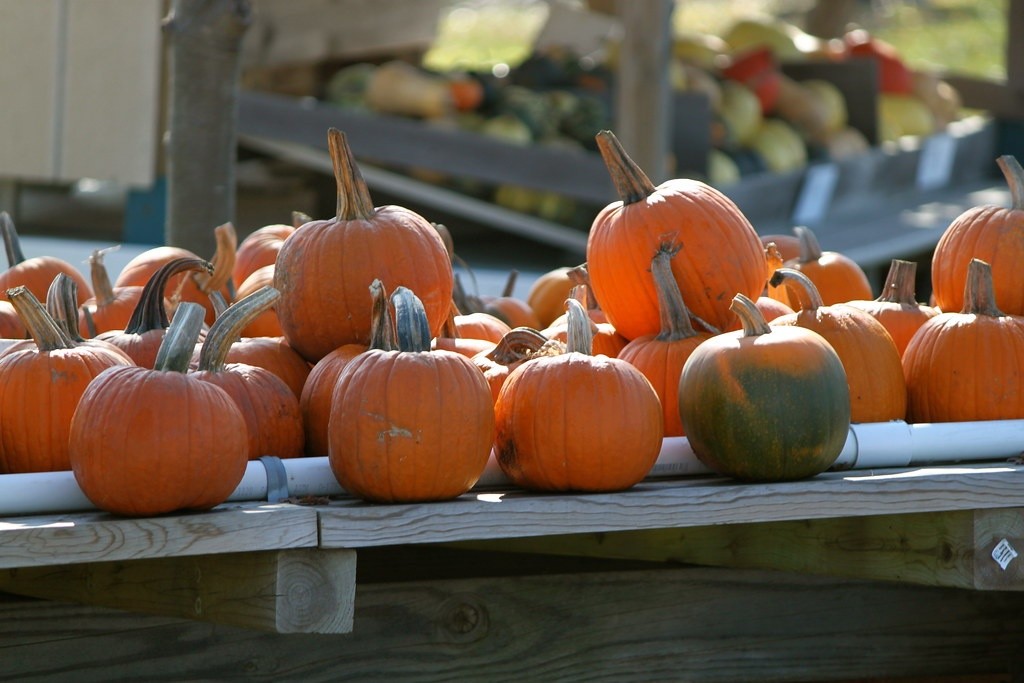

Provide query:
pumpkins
left=0, top=14, right=1024, bottom=514
